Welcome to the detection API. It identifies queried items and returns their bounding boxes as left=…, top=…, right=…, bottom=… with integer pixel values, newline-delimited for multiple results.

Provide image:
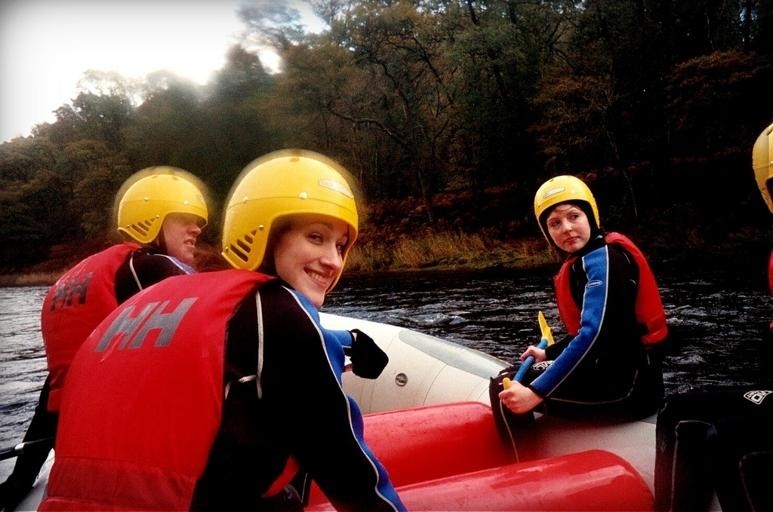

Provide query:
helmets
left=117, top=172, right=209, bottom=244
left=531, top=174, right=601, bottom=247
left=751, top=124, right=772, bottom=212
left=221, top=156, right=361, bottom=296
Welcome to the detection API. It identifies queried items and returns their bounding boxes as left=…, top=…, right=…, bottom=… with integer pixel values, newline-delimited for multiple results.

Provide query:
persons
left=39, top=149, right=406, bottom=512
left=490, top=176, right=667, bottom=466
left=0, top=165, right=388, bottom=511
left=653, top=121, right=773, bottom=512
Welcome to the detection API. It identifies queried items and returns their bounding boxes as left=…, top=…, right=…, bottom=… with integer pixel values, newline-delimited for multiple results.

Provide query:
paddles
left=503, top=309, right=554, bottom=390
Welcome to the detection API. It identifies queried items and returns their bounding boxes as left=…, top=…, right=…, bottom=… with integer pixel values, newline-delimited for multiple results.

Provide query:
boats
left=0, top=311, right=720, bottom=512
